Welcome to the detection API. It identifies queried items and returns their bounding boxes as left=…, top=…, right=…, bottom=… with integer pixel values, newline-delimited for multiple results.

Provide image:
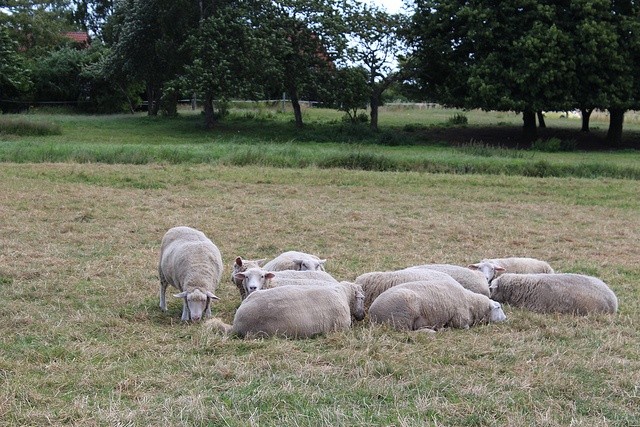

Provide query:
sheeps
left=231, top=256, right=267, bottom=302
left=368, top=280, right=507, bottom=334
left=489, top=273, right=619, bottom=314
left=261, top=250, right=327, bottom=272
left=403, top=264, right=492, bottom=298
left=468, top=257, right=555, bottom=291
left=232, top=281, right=366, bottom=341
left=353, top=268, right=465, bottom=311
left=157, top=226, right=223, bottom=322
left=234, top=268, right=340, bottom=297
